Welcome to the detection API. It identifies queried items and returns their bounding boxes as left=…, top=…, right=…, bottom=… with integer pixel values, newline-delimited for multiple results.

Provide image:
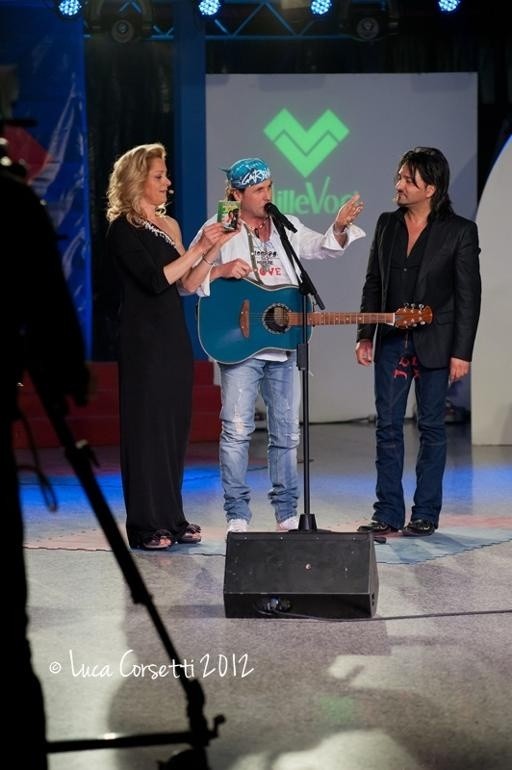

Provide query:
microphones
left=168, top=189, right=175, bottom=194
left=265, top=203, right=298, bottom=233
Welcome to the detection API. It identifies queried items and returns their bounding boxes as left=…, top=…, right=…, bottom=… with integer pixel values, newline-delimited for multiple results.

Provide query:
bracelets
left=203, top=258, right=214, bottom=266
left=334, top=227, right=347, bottom=236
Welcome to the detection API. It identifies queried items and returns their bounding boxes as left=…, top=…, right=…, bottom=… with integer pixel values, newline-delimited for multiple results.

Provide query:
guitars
left=196, top=278, right=433, bottom=364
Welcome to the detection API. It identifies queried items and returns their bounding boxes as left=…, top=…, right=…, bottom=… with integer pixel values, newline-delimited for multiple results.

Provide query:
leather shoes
left=357, top=521, right=398, bottom=534
left=403, top=520, right=434, bottom=536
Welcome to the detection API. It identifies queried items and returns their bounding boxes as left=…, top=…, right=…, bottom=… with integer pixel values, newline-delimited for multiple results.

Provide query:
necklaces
left=245, top=218, right=269, bottom=238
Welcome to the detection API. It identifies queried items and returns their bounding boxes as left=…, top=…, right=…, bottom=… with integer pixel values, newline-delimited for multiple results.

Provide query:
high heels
left=178, top=524, right=201, bottom=544
left=142, top=530, right=174, bottom=550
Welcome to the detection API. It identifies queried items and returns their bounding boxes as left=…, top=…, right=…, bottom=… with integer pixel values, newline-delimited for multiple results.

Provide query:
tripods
left=0, top=163, right=215, bottom=770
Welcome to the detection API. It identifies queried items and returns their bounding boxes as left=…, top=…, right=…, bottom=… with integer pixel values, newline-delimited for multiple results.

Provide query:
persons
left=105, top=143, right=243, bottom=551
left=356, top=146, right=482, bottom=538
left=0, top=153, right=91, bottom=770
left=175, top=158, right=366, bottom=541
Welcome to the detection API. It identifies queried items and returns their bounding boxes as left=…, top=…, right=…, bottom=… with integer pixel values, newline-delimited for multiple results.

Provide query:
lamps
left=333, top=0, right=401, bottom=43
left=88, top=2, right=153, bottom=48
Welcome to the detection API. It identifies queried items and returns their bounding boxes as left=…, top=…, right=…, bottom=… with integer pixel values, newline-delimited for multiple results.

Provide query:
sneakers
left=279, top=517, right=299, bottom=532
left=225, top=518, right=248, bottom=541
